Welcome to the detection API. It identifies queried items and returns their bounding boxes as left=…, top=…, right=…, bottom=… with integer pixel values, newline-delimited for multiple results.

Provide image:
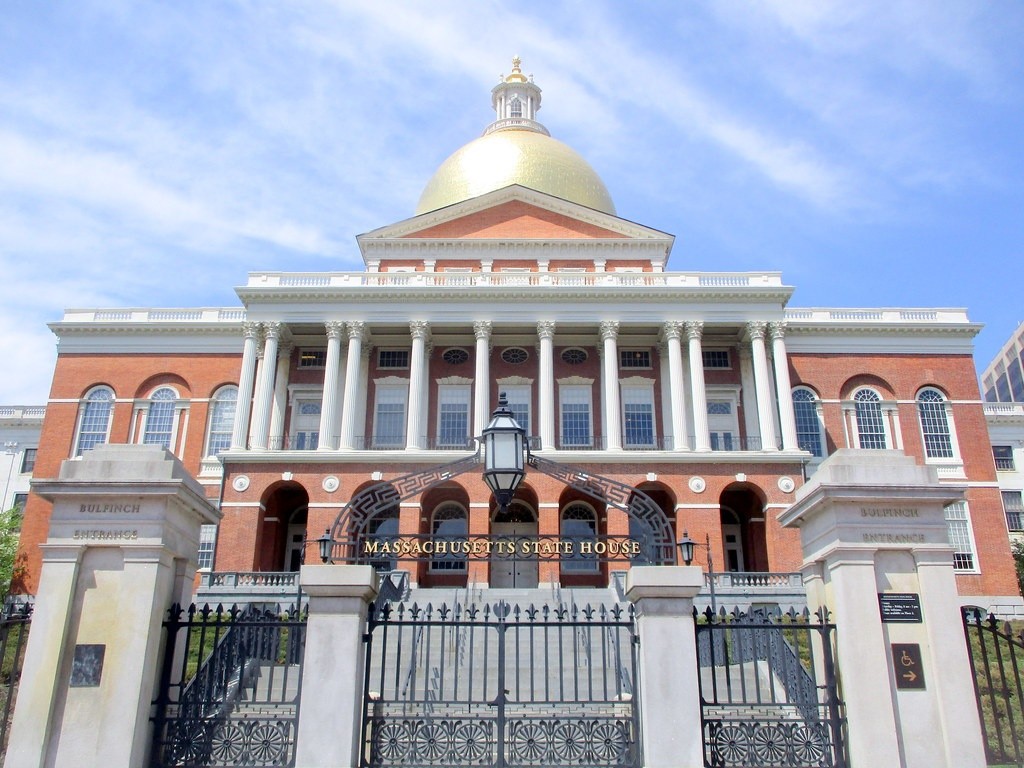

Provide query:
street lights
left=482, top=392, right=529, bottom=509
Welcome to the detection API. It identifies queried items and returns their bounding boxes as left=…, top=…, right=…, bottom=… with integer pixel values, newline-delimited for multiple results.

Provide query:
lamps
left=318, top=525, right=336, bottom=564
left=676, top=529, right=696, bottom=567
